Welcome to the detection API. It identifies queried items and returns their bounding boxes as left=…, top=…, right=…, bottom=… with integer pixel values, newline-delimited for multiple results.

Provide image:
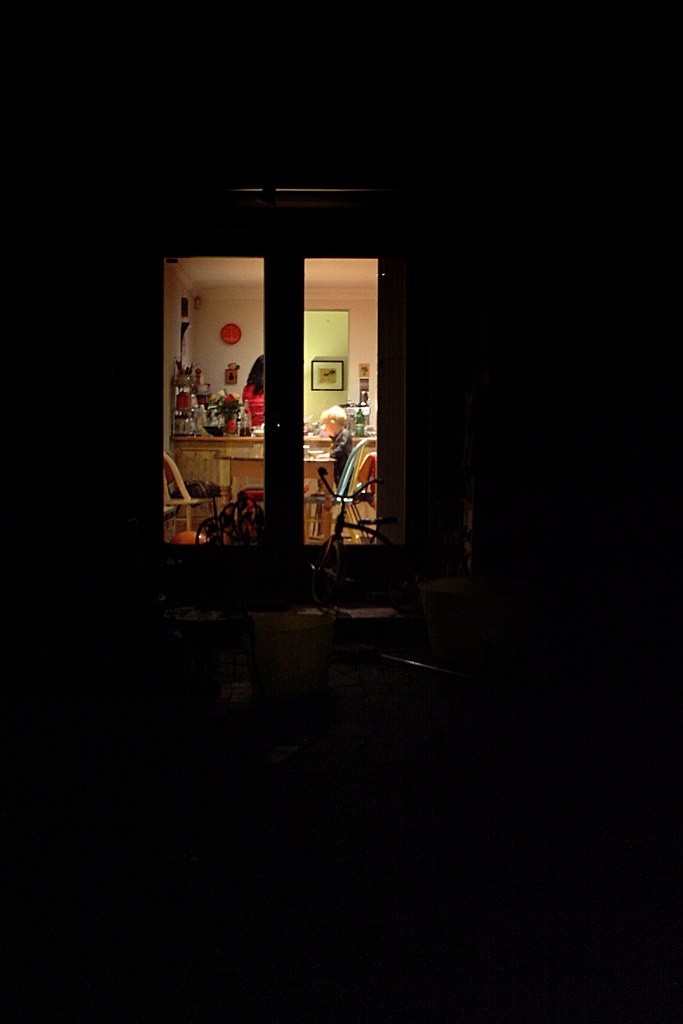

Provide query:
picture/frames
left=311, top=360, right=344, bottom=391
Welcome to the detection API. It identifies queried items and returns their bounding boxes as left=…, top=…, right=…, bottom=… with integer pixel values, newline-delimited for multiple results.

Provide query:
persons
left=311, top=404, right=353, bottom=496
left=242, top=353, right=264, bottom=427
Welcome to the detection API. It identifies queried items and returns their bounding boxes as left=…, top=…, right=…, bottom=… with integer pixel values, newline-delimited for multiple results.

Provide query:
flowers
left=207, top=389, right=249, bottom=421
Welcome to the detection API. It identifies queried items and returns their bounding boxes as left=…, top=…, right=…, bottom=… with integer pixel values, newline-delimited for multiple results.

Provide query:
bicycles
left=311, top=467, right=438, bottom=613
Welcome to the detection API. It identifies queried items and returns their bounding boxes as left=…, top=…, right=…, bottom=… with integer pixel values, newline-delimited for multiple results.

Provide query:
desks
left=214, top=450, right=337, bottom=539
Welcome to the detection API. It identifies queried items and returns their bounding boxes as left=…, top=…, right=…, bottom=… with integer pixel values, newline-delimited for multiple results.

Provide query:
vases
left=226, top=414, right=237, bottom=435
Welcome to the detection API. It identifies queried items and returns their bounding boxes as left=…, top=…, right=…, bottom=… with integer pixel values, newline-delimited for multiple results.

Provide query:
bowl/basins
left=308, top=451, right=324, bottom=458
left=253, top=431, right=264, bottom=437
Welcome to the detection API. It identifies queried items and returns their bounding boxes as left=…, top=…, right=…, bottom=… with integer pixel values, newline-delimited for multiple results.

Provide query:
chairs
left=164, top=451, right=217, bottom=533
left=305, top=437, right=369, bottom=538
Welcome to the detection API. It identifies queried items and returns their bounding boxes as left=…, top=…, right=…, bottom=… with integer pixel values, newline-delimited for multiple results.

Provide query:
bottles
left=355, top=409, right=364, bottom=437
left=236, top=409, right=241, bottom=436
left=240, top=400, right=251, bottom=436
left=191, top=369, right=202, bottom=393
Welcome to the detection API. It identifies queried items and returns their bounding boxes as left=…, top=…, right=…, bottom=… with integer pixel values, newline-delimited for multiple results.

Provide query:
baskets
left=165, top=579, right=485, bottom=684
